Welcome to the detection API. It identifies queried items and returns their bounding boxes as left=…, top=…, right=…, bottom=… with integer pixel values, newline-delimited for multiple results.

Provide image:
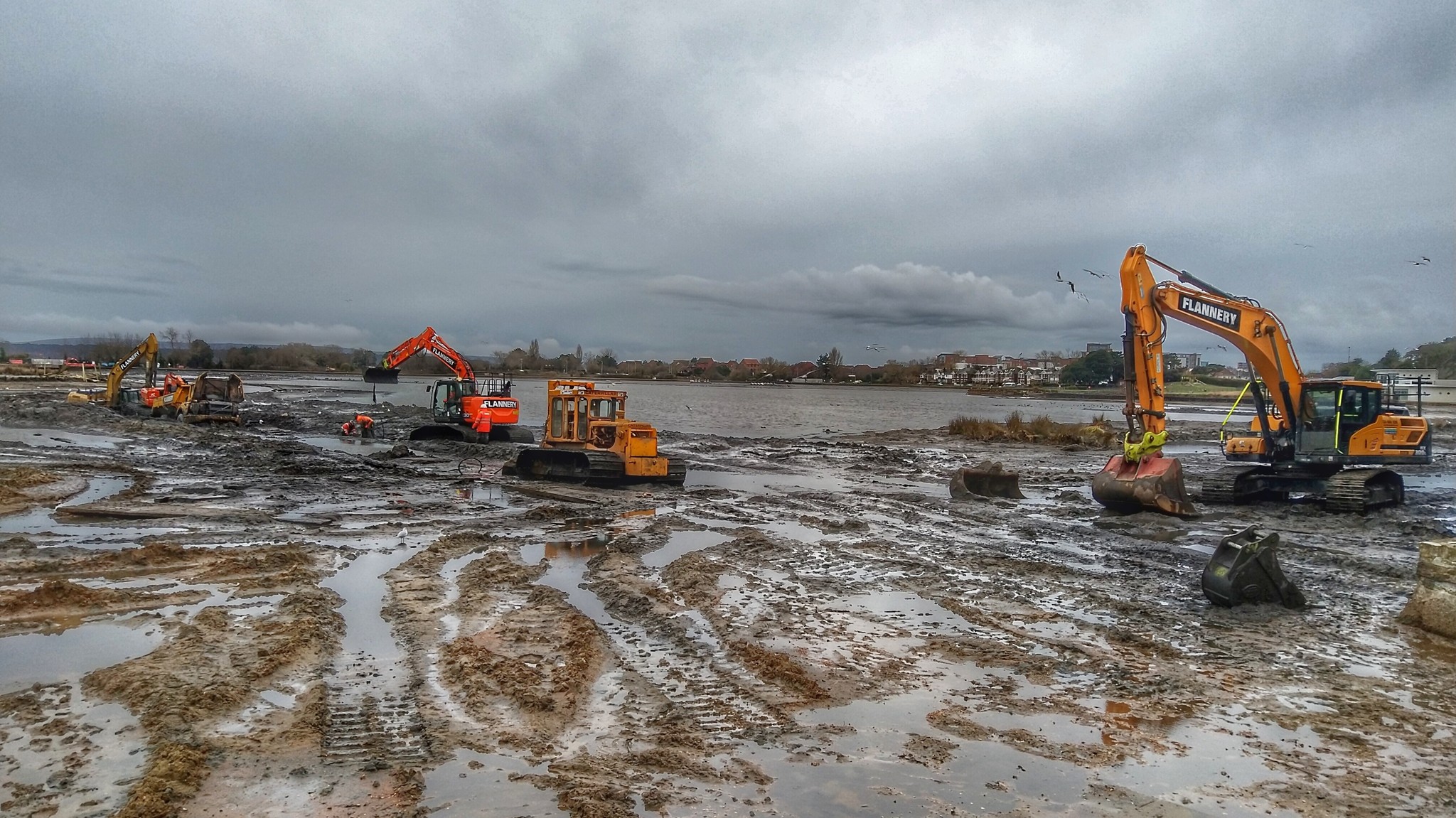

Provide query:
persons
left=353, top=412, right=374, bottom=438
left=342, top=420, right=356, bottom=436
left=443, top=390, right=455, bottom=402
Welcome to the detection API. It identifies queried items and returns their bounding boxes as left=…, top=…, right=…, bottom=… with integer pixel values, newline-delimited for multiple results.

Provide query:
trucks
left=6, top=359, right=23, bottom=365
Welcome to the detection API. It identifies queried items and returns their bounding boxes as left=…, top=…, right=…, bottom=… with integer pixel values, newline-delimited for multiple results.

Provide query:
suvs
left=157, top=362, right=160, bottom=368
left=108, top=362, right=115, bottom=367
left=101, top=363, right=108, bottom=367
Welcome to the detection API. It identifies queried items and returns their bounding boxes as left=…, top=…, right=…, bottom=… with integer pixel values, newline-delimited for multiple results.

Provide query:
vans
left=1002, top=383, right=1015, bottom=388
left=329, top=368, right=336, bottom=372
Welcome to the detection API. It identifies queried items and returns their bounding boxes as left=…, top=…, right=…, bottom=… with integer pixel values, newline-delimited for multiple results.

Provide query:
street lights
left=566, top=361, right=567, bottom=373
left=628, top=362, right=630, bottom=374
left=871, top=375, right=872, bottom=381
left=1348, top=347, right=1350, bottom=362
left=601, top=361, right=602, bottom=374
left=520, top=359, right=523, bottom=370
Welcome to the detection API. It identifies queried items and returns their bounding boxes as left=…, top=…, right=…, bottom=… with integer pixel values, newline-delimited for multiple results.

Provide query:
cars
left=966, top=384, right=973, bottom=386
left=937, top=383, right=943, bottom=385
left=940, top=382, right=946, bottom=384
left=916, top=382, right=922, bottom=385
left=1017, top=383, right=1022, bottom=386
left=839, top=381, right=845, bottom=384
left=1099, top=381, right=1108, bottom=385
left=853, top=380, right=863, bottom=384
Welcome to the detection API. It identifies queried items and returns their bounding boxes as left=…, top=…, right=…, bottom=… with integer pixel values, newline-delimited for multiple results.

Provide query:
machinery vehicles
left=67, top=333, right=245, bottom=424
left=502, top=379, right=686, bottom=488
left=1091, top=243, right=1432, bottom=519
left=325, top=365, right=330, bottom=372
left=363, top=327, right=535, bottom=445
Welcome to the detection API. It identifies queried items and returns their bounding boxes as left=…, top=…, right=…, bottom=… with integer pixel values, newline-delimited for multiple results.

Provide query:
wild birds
left=863, top=344, right=886, bottom=352
left=1206, top=345, right=1227, bottom=352
left=1404, top=344, right=1429, bottom=350
left=258, top=390, right=413, bottom=424
left=1056, top=269, right=1116, bottom=304
left=686, top=405, right=694, bottom=410
left=1294, top=243, right=1314, bottom=248
left=606, top=380, right=622, bottom=386
left=393, top=527, right=408, bottom=543
left=962, top=454, right=968, bottom=459
left=1407, top=256, right=1431, bottom=265
